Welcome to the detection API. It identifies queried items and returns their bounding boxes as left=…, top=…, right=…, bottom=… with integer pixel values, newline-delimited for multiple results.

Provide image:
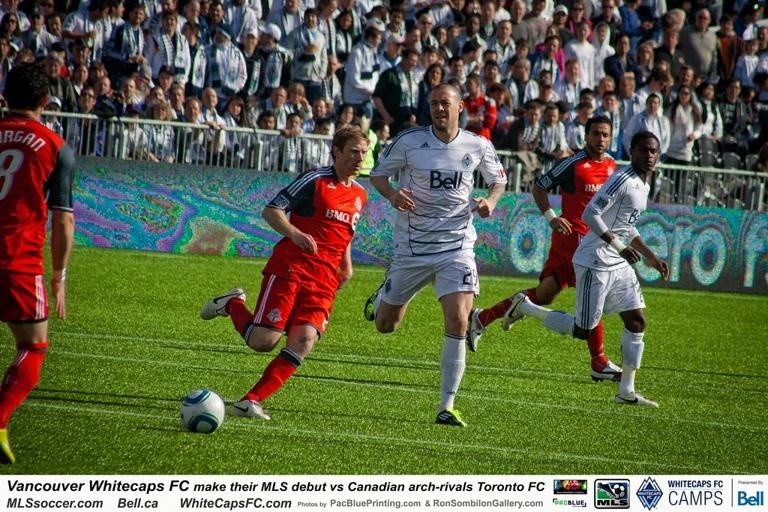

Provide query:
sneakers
left=615, top=393, right=659, bottom=409
left=201, top=289, right=246, bottom=320
left=364, top=283, right=385, bottom=321
left=467, top=307, right=486, bottom=352
left=435, top=407, right=467, bottom=427
left=501, top=293, right=530, bottom=331
left=233, top=399, right=271, bottom=420
left=0, top=428, right=16, bottom=464
left=591, top=361, right=622, bottom=382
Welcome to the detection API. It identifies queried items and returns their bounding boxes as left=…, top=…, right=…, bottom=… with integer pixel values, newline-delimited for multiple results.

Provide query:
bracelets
left=610, top=238, right=626, bottom=254
left=543, top=208, right=556, bottom=222
left=50, top=267, right=67, bottom=282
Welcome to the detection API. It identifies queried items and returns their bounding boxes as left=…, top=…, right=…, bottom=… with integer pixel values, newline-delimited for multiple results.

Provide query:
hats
left=53, top=42, right=68, bottom=52
left=44, top=97, right=62, bottom=108
left=259, top=23, right=282, bottom=41
left=127, top=103, right=140, bottom=114
left=158, top=64, right=175, bottom=76
left=244, top=28, right=258, bottom=38
left=217, top=24, right=234, bottom=40
left=554, top=5, right=569, bottom=16
left=28, top=4, right=45, bottom=16
left=68, top=37, right=91, bottom=53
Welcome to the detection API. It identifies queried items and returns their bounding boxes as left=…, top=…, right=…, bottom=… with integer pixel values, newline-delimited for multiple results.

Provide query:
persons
left=365, top=84, right=508, bottom=427
left=465, top=116, right=627, bottom=382
left=0, top=62, right=76, bottom=463
left=501, top=131, right=670, bottom=409
left=201, top=128, right=370, bottom=420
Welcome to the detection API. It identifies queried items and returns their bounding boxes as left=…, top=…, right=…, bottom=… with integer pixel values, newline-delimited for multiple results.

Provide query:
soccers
left=180, top=389, right=225, bottom=433
